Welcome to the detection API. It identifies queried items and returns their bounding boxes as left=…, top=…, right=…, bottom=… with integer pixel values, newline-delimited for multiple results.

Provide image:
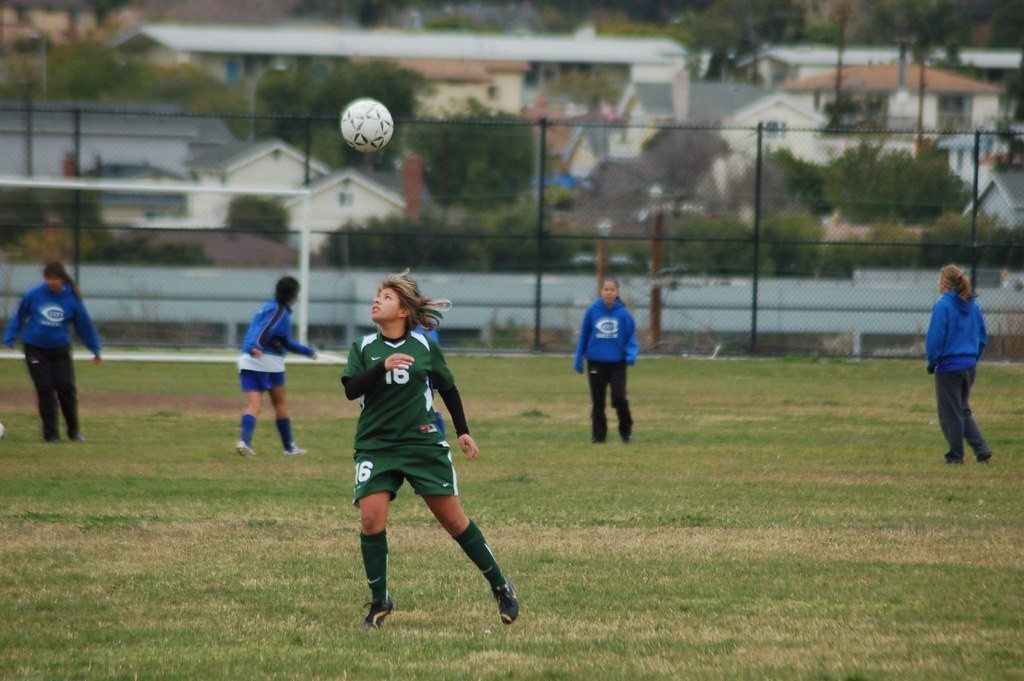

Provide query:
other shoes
left=71, top=434, right=86, bottom=442
left=46, top=438, right=61, bottom=443
left=284, top=442, right=307, bottom=455
left=237, top=441, right=257, bottom=456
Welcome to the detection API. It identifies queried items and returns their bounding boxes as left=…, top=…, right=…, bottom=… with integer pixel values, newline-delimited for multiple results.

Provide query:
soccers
left=340, top=98, right=395, bottom=154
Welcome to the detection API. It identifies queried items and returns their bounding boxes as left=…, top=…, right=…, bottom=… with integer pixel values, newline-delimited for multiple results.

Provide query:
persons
left=574, top=278, right=640, bottom=444
left=236, top=276, right=317, bottom=457
left=3, top=260, right=102, bottom=444
left=341, top=268, right=520, bottom=632
left=924, top=263, right=993, bottom=464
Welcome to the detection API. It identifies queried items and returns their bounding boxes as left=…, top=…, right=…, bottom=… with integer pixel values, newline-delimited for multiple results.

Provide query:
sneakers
left=492, top=578, right=519, bottom=624
left=364, top=596, right=393, bottom=630
